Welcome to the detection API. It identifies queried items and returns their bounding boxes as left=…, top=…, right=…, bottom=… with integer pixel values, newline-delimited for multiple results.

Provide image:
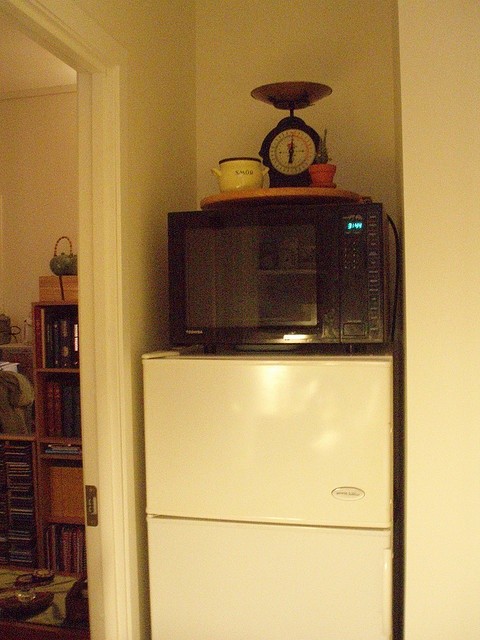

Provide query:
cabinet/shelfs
left=33, top=299, right=85, bottom=578
left=1, top=436, right=32, bottom=566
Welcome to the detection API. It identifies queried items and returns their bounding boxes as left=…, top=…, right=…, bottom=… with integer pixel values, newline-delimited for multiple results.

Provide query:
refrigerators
left=142, top=352, right=392, bottom=640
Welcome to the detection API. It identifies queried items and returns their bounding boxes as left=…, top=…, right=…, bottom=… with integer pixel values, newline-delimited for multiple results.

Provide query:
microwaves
left=168, top=202, right=392, bottom=352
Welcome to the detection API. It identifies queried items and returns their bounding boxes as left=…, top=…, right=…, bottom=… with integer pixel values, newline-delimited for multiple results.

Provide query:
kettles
left=49, top=236, right=77, bottom=276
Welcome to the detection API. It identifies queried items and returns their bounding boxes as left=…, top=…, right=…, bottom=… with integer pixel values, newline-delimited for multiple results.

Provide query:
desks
left=1, top=564, right=89, bottom=638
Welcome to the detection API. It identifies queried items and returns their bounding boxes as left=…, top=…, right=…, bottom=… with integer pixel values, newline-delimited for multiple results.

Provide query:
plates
left=201, top=187, right=362, bottom=208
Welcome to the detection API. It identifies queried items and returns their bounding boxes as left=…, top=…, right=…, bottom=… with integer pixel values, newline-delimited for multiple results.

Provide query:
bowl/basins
left=211, top=157, right=270, bottom=192
